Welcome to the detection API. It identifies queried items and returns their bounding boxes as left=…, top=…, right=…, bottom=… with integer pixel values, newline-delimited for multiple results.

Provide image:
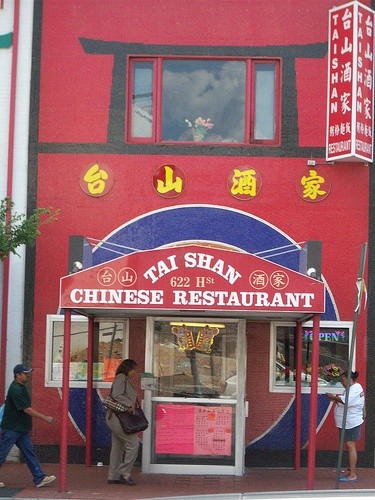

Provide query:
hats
left=13, top=364, right=33, bottom=373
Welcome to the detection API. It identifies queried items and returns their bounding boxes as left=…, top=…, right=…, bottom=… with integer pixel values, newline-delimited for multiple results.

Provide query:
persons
left=105, top=358, right=137, bottom=486
left=326, top=370, right=365, bottom=483
left=0, top=364, right=56, bottom=487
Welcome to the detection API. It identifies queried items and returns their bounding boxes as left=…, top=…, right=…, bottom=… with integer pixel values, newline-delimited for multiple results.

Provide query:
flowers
left=322, top=363, right=344, bottom=385
left=184, top=117, right=215, bottom=140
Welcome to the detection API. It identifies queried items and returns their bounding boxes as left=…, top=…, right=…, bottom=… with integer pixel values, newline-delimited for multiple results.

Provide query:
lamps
left=307, top=267, right=317, bottom=279
left=72, top=261, right=83, bottom=274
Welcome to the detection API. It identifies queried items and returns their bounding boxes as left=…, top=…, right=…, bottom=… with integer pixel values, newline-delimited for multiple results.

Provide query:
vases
left=193, top=134, right=202, bottom=140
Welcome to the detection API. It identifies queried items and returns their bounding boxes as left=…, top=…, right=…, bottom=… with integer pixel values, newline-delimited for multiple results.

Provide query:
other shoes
left=107, top=480, right=127, bottom=484
left=121, top=474, right=136, bottom=485
left=36, top=475, right=57, bottom=488
left=339, top=475, right=358, bottom=483
left=0, top=482, right=4, bottom=488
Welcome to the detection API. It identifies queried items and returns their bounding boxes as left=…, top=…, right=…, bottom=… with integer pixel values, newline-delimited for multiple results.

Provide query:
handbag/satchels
left=115, top=407, right=148, bottom=435
left=102, top=397, right=130, bottom=413
